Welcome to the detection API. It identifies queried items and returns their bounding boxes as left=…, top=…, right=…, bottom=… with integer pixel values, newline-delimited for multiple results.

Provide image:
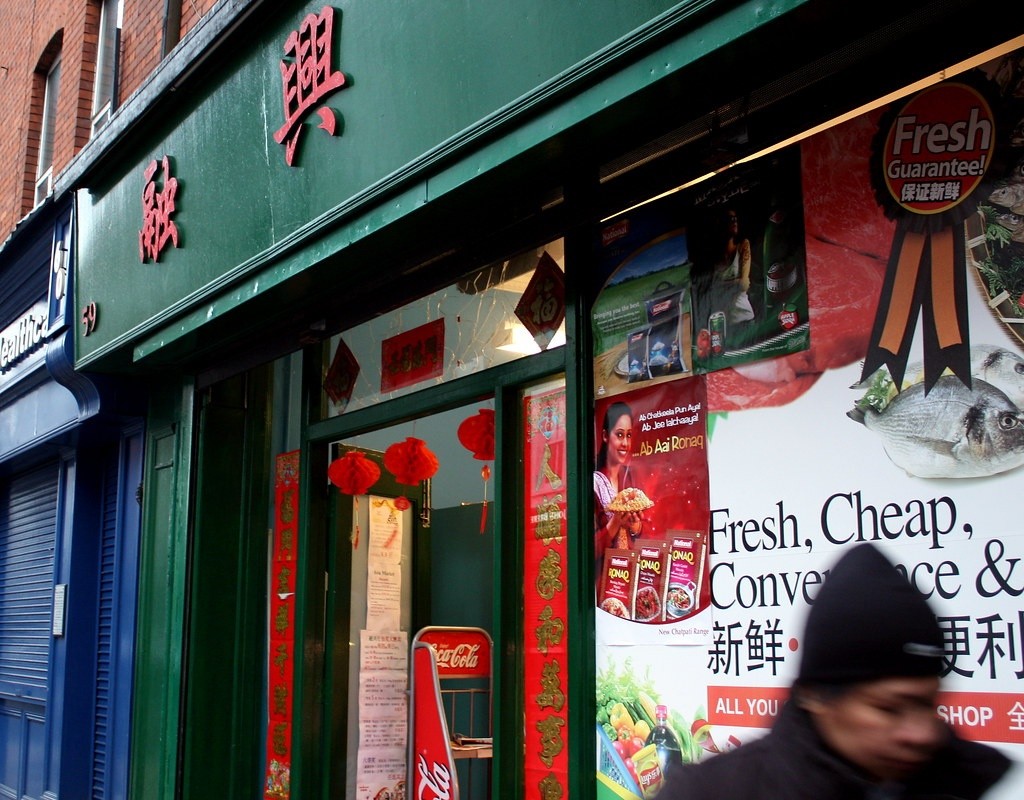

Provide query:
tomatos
left=610, top=738, right=644, bottom=783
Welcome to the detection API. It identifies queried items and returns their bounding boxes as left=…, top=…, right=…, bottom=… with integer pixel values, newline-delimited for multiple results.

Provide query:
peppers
left=597, top=700, right=649, bottom=746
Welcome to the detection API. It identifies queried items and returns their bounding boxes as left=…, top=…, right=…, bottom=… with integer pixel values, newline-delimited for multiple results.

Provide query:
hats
left=799, top=544, right=946, bottom=684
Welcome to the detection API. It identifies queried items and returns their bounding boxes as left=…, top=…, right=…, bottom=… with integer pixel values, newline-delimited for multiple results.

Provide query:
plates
left=881, top=440, right=1024, bottom=480
left=614, top=353, right=629, bottom=376
left=608, top=501, right=654, bottom=514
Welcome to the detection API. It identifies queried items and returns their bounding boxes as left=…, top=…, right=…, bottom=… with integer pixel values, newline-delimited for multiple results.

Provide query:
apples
left=697, top=329, right=711, bottom=359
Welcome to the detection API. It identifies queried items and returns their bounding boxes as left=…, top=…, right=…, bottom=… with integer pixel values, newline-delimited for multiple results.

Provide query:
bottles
left=644, top=705, right=683, bottom=784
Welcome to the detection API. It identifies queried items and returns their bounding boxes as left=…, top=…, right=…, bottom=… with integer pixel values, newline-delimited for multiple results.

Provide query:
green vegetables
left=595, top=655, right=660, bottom=706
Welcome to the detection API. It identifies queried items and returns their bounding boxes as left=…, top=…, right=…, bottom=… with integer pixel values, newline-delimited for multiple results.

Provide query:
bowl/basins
left=766, top=265, right=797, bottom=293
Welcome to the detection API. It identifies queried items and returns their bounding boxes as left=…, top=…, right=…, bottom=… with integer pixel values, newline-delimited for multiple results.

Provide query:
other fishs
left=847, top=345, right=1023, bottom=479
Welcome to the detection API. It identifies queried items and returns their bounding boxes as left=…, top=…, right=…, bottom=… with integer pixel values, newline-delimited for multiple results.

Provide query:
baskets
left=597, top=721, right=642, bottom=798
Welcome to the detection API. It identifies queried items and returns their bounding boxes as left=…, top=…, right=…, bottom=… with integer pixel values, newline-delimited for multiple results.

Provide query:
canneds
left=707, top=312, right=726, bottom=357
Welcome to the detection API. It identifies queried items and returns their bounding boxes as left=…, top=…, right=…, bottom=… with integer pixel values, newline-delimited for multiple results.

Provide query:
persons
left=650, top=541, right=1024, bottom=800
left=695, top=204, right=755, bottom=359
left=592, top=400, right=657, bottom=606
